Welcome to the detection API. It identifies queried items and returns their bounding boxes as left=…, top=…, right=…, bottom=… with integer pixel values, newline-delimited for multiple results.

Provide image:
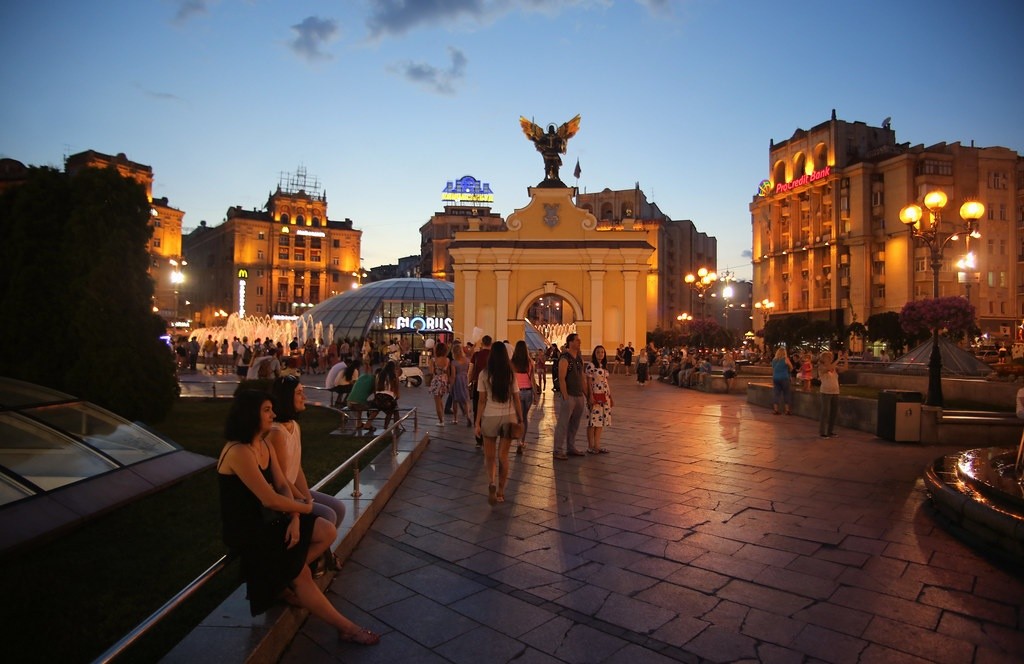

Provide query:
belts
left=519, top=387, right=533, bottom=391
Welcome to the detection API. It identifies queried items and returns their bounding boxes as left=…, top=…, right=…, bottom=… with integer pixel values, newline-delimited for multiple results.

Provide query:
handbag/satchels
left=468, top=380, right=476, bottom=400
left=504, top=421, right=523, bottom=440
left=593, top=392, right=607, bottom=405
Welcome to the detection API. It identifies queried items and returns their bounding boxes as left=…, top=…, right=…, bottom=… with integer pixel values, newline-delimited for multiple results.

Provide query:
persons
left=430, top=333, right=613, bottom=459
left=347, top=368, right=383, bottom=432
left=473, top=342, right=525, bottom=506
left=266, top=375, right=344, bottom=578
left=817, top=350, right=850, bottom=439
left=861, top=348, right=890, bottom=366
left=999, top=346, right=1006, bottom=364
left=721, top=353, right=737, bottom=393
left=326, top=358, right=360, bottom=407
left=245, top=348, right=281, bottom=393
left=375, top=361, right=400, bottom=430
left=772, top=347, right=816, bottom=416
left=612, top=340, right=711, bottom=390
left=170, top=335, right=411, bottom=375
left=216, top=391, right=380, bottom=645
left=535, top=125, right=563, bottom=181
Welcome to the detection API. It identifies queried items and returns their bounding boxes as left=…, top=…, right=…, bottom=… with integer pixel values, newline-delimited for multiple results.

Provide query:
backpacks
left=257, top=357, right=277, bottom=380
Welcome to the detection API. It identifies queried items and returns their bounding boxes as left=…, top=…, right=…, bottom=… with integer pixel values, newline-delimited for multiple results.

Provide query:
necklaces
left=284, top=422, right=292, bottom=433
left=252, top=440, right=264, bottom=456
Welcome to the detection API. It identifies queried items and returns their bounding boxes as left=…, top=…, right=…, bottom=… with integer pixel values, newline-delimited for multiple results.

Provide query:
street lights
left=169, top=253, right=189, bottom=335
left=352, top=272, right=368, bottom=287
left=722, top=287, right=733, bottom=329
left=755, top=298, right=775, bottom=327
left=684, top=267, right=718, bottom=347
left=677, top=313, right=693, bottom=334
left=897, top=191, right=988, bottom=406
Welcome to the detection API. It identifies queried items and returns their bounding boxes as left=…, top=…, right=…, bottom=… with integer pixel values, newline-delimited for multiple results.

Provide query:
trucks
left=1012, top=342, right=1024, bottom=360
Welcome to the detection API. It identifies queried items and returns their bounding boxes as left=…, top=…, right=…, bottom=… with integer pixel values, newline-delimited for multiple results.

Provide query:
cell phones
left=840, top=351, right=842, bottom=358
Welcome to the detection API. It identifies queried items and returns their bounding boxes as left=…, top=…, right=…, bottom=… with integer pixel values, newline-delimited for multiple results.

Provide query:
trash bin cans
left=877, top=389, right=922, bottom=443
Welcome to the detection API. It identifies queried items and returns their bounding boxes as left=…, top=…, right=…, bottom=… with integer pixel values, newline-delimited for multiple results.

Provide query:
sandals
left=496, top=491, right=505, bottom=503
left=772, top=410, right=782, bottom=415
left=784, top=410, right=792, bottom=415
left=488, top=482, right=497, bottom=506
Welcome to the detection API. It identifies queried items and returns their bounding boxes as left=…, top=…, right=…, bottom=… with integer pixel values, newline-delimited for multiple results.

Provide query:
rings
left=295, top=539, right=297, bottom=542
left=291, top=538, right=295, bottom=541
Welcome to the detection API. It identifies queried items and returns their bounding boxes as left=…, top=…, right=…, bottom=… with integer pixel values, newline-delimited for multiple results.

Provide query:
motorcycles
left=375, top=354, right=423, bottom=388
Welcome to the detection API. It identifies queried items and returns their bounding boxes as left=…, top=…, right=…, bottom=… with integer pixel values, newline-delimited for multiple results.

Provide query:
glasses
left=282, top=375, right=297, bottom=386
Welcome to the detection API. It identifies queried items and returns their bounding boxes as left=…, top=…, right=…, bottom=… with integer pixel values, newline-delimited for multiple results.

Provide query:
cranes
left=719, top=262, right=753, bottom=282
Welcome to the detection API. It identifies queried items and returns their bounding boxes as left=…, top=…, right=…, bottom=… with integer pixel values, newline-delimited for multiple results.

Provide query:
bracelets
left=519, top=421, right=524, bottom=422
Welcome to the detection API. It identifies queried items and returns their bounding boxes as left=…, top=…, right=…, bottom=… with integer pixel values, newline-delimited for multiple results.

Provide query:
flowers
left=687, top=319, right=720, bottom=336
left=898, top=296, right=983, bottom=345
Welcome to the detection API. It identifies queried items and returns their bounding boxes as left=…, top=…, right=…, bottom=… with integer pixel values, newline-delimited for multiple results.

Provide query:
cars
left=975, top=349, right=999, bottom=364
left=989, top=357, right=1024, bottom=377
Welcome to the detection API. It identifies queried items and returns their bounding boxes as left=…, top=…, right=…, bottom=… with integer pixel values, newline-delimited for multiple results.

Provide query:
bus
left=983, top=345, right=1007, bottom=350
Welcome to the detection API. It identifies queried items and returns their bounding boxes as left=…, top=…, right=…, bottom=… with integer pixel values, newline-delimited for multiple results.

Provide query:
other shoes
left=435, top=422, right=444, bottom=427
left=450, top=419, right=459, bottom=425
left=587, top=447, right=610, bottom=455
left=819, top=434, right=830, bottom=440
left=517, top=442, right=526, bottom=455
left=553, top=452, right=568, bottom=460
left=475, top=442, right=482, bottom=448
left=309, top=551, right=343, bottom=580
left=637, top=380, right=643, bottom=386
left=827, top=433, right=839, bottom=438
left=444, top=410, right=453, bottom=414
left=567, top=449, right=585, bottom=456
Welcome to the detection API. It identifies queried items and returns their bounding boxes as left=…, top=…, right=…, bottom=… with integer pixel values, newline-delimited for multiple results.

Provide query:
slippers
left=365, top=426, right=377, bottom=431
left=361, top=422, right=364, bottom=428
left=284, top=591, right=305, bottom=609
left=337, top=627, right=379, bottom=646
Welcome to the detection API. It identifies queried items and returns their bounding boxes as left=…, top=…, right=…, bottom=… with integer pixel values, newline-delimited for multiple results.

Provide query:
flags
left=574, top=161, right=581, bottom=178
